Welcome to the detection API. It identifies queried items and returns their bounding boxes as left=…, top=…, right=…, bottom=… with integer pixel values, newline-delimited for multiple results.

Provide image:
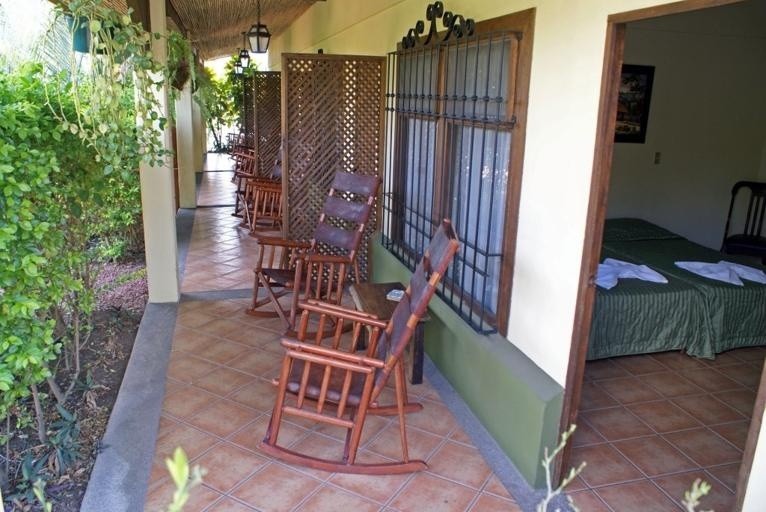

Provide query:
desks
left=348, top=282, right=430, bottom=385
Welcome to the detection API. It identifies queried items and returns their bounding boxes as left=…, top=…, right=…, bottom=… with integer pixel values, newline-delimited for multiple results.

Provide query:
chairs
left=236, top=139, right=307, bottom=239
left=257, top=220, right=459, bottom=473
left=245, top=165, right=384, bottom=343
left=720, top=181, right=766, bottom=267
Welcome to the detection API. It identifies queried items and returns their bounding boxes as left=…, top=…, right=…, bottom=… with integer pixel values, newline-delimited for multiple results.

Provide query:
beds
left=586, top=219, right=765, bottom=360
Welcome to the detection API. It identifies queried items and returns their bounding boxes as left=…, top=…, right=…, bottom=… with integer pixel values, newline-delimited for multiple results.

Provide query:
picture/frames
left=613, top=64, right=656, bottom=144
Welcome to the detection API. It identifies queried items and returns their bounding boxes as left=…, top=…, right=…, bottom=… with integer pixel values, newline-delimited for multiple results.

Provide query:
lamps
left=234, top=1, right=271, bottom=78
